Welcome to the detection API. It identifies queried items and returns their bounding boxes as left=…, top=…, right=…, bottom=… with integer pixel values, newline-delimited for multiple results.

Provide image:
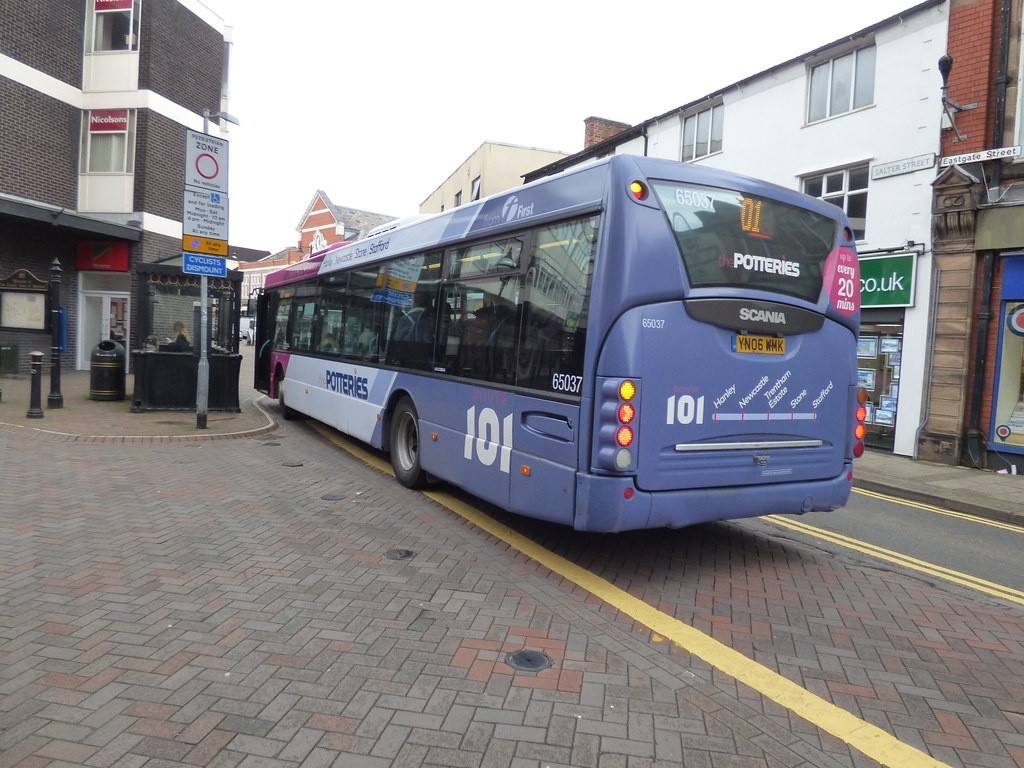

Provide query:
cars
left=239, top=305, right=256, bottom=344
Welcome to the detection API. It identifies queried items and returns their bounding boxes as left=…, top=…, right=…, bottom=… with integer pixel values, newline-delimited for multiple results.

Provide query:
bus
left=247, top=154, right=871, bottom=534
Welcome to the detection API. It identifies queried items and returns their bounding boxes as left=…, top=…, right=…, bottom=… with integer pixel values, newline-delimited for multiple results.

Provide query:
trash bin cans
left=89, top=340, right=126, bottom=402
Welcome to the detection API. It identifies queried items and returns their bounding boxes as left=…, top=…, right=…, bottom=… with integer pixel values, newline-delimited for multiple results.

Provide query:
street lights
left=194, top=108, right=241, bottom=429
left=46, top=256, right=65, bottom=409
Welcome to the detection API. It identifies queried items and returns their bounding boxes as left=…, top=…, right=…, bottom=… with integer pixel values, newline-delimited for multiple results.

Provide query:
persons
left=166, top=322, right=191, bottom=352
left=316, top=289, right=455, bottom=356
